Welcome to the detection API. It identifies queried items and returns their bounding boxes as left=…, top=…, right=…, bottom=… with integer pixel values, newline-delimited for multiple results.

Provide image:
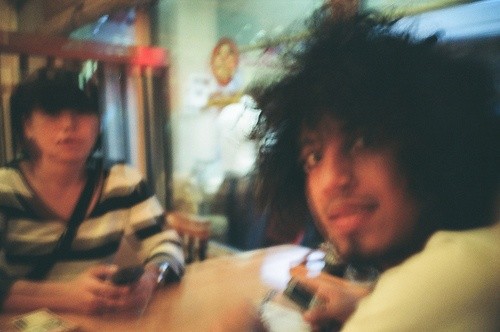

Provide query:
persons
left=0, top=65, right=191, bottom=328
left=207, top=118, right=324, bottom=257
left=230, top=1, right=500, bottom=332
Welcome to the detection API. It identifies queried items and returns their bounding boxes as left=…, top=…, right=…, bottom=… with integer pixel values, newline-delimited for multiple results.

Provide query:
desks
left=1, top=241, right=363, bottom=332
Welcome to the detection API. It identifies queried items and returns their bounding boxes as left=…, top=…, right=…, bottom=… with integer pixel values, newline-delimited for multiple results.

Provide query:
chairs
left=164, top=211, right=212, bottom=265
